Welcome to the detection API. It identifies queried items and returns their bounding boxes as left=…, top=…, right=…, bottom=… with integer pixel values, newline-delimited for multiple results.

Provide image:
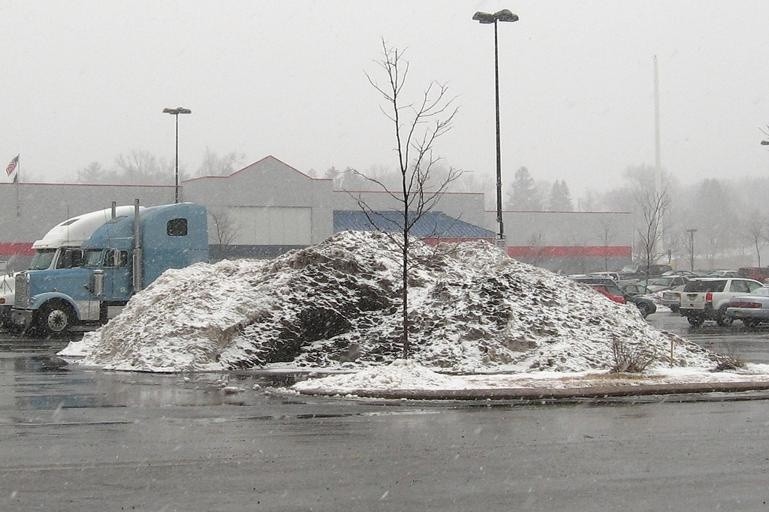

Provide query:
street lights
left=162, top=105, right=190, bottom=202
left=471, top=8, right=519, bottom=240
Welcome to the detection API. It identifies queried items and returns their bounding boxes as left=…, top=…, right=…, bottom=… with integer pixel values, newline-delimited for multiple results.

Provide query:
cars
left=567, top=262, right=768, bottom=331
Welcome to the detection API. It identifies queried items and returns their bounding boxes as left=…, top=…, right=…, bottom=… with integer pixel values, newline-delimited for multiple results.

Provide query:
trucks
left=9, top=201, right=209, bottom=341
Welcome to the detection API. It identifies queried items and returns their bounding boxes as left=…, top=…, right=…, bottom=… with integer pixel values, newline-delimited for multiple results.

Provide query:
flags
left=5, top=154, right=19, bottom=177
